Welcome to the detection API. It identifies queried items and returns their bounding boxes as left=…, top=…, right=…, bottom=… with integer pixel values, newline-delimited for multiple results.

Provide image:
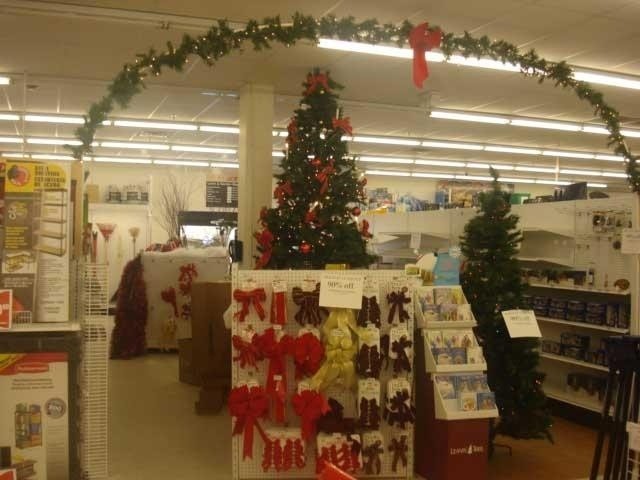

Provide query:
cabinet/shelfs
left=378, top=228, right=630, bottom=417
left=84, top=174, right=152, bottom=309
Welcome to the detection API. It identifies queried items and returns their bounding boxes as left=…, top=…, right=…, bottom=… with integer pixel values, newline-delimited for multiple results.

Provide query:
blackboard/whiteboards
left=206, top=181, right=238, bottom=207
